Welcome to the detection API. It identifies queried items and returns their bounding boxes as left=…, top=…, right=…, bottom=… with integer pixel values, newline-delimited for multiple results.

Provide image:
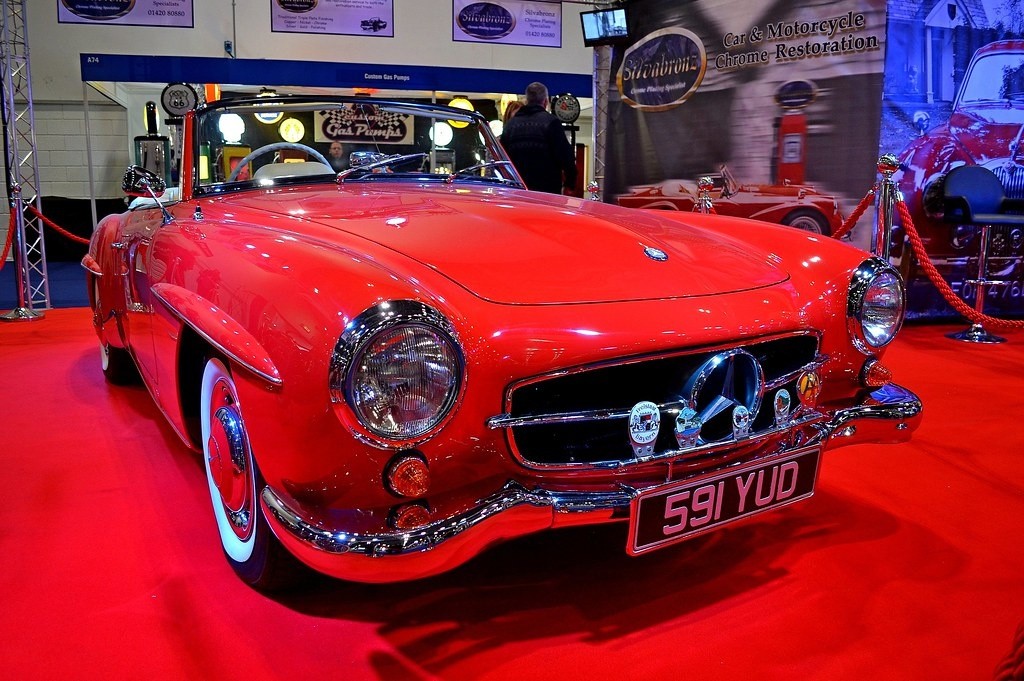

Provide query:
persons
left=324, top=141, right=350, bottom=173
left=484, top=81, right=577, bottom=196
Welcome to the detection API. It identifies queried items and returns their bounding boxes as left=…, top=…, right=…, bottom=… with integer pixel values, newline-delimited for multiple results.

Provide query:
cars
left=81, top=95, right=923, bottom=601
left=881, top=38, right=1024, bottom=325
left=615, top=161, right=850, bottom=245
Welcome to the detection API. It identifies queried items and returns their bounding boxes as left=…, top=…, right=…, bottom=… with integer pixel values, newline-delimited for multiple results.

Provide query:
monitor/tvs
left=579, top=7, right=633, bottom=47
